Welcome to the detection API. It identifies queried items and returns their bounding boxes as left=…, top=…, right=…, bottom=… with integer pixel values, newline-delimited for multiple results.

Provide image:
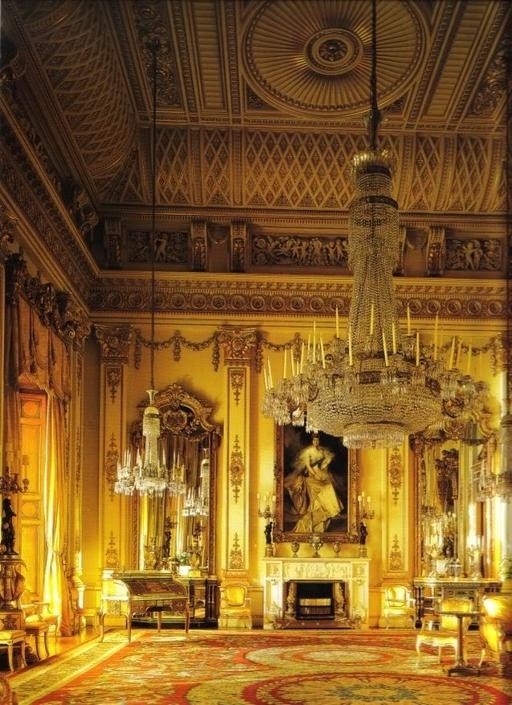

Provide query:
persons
left=234, top=242, right=244, bottom=272
left=253, top=235, right=349, bottom=265
left=132, top=237, right=181, bottom=263
left=195, top=241, right=206, bottom=271
left=291, top=433, right=345, bottom=533
left=448, top=241, right=502, bottom=271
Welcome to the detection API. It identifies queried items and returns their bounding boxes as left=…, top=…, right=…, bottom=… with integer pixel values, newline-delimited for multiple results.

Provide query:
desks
left=414, top=577, right=503, bottom=630
left=435, top=611, right=486, bottom=677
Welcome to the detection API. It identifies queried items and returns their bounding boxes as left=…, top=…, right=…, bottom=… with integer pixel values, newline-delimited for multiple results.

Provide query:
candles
left=257, top=491, right=276, bottom=502
left=358, top=491, right=372, bottom=503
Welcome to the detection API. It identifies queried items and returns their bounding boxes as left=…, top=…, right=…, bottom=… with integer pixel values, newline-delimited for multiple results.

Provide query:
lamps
left=181, top=438, right=209, bottom=516
left=115, top=1, right=186, bottom=497
left=261, top=1, right=502, bottom=450
left=0, top=442, right=30, bottom=560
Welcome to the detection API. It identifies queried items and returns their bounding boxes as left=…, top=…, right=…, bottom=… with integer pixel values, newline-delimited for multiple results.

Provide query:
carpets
left=0, top=630, right=512, bottom=705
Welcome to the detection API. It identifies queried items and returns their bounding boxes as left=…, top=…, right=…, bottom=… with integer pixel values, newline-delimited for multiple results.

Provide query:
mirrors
left=125, top=382, right=220, bottom=628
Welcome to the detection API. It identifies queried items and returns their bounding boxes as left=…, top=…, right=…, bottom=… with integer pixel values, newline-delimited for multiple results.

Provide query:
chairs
left=0, top=619, right=28, bottom=672
left=416, top=597, right=475, bottom=668
left=382, top=582, right=416, bottom=630
left=218, top=584, right=252, bottom=630
left=18, top=599, right=49, bottom=660
left=477, top=592, right=512, bottom=678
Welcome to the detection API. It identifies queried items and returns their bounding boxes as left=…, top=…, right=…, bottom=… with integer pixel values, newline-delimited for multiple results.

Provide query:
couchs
left=20, top=588, right=58, bottom=643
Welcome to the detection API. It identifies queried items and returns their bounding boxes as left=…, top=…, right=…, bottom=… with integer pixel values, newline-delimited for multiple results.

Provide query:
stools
left=73, top=607, right=100, bottom=635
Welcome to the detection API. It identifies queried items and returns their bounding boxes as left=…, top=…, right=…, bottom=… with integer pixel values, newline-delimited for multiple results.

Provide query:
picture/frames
left=273, top=417, right=360, bottom=543
